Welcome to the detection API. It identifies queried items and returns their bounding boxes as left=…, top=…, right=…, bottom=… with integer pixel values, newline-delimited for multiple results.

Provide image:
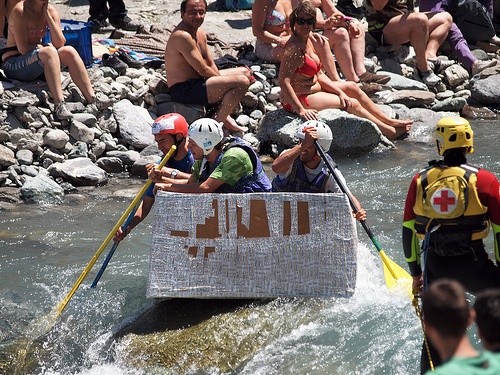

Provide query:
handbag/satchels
left=41, top=18, right=94, bottom=65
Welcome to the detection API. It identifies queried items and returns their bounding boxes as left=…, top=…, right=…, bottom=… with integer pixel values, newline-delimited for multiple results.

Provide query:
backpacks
left=456, top=0, right=495, bottom=42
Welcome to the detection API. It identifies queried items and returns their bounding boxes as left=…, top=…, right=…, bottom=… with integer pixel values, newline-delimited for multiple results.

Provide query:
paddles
left=312, top=139, right=414, bottom=302
left=94, top=179, right=157, bottom=286
left=44, top=145, right=178, bottom=332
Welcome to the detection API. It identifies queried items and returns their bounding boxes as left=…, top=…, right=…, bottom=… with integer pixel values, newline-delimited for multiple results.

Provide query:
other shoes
left=488, top=34, right=500, bottom=45
left=54, top=101, right=74, bottom=119
left=472, top=59, right=497, bottom=75
left=88, top=17, right=110, bottom=28
left=358, top=71, right=392, bottom=85
left=101, top=53, right=128, bottom=71
left=364, top=56, right=382, bottom=74
left=427, top=58, right=460, bottom=74
left=354, top=80, right=384, bottom=95
left=91, top=94, right=116, bottom=110
left=414, top=66, right=442, bottom=85
left=115, top=49, right=144, bottom=67
left=111, top=15, right=145, bottom=30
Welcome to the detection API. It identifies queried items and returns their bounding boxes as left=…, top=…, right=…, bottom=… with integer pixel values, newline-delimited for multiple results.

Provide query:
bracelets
left=171, top=169, right=179, bottom=179
left=345, top=21, right=349, bottom=28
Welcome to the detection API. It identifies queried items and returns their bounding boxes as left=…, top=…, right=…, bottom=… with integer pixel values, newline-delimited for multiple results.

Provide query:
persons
left=422, top=279, right=500, bottom=375
left=270, top=119, right=366, bottom=221
left=113, top=113, right=273, bottom=245
left=252, top=0, right=414, bottom=139
left=403, top=117, right=500, bottom=375
left=0, top=0, right=116, bottom=119
left=165, top=0, right=250, bottom=132
left=86, top=0, right=142, bottom=31
left=362, top=0, right=500, bottom=85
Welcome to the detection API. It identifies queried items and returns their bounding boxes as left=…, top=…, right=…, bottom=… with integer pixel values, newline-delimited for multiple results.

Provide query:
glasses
left=294, top=11, right=316, bottom=25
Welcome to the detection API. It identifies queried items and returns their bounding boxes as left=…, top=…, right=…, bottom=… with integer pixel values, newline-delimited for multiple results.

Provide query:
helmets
left=296, top=119, right=332, bottom=156
left=188, top=118, right=224, bottom=156
left=434, top=115, right=474, bottom=155
left=152, top=113, right=187, bottom=142
